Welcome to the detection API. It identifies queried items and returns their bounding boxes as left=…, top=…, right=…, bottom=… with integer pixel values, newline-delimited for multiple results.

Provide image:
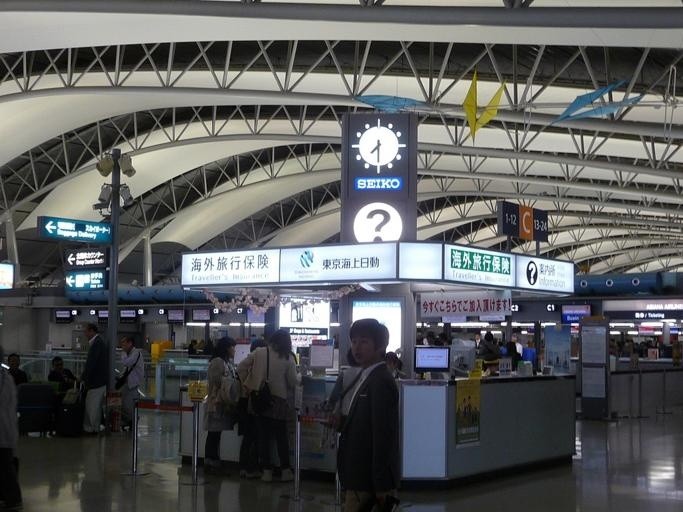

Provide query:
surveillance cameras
left=93, top=203, right=109, bottom=209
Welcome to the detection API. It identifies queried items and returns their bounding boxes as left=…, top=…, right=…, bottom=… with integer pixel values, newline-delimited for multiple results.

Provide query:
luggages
left=55, top=391, right=86, bottom=438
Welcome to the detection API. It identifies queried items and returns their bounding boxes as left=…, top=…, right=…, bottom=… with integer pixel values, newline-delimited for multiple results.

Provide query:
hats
left=482, top=355, right=500, bottom=367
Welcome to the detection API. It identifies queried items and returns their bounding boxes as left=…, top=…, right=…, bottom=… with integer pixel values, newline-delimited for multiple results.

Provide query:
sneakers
left=239, top=469, right=246, bottom=481
left=280, top=468, right=295, bottom=482
left=80, top=431, right=98, bottom=438
left=247, top=471, right=262, bottom=482
left=0, top=500, right=24, bottom=511
left=261, top=468, right=274, bottom=484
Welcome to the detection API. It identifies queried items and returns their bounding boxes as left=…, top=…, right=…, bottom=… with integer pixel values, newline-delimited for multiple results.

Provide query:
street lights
left=91, top=149, right=135, bottom=393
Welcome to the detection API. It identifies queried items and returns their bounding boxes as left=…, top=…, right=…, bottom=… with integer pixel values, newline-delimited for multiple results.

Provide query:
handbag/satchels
left=220, top=374, right=242, bottom=404
left=249, top=381, right=276, bottom=415
left=114, top=366, right=130, bottom=391
left=219, top=423, right=245, bottom=464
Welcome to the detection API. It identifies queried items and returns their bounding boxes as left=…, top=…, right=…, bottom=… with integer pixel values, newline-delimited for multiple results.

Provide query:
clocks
left=348, top=115, right=408, bottom=176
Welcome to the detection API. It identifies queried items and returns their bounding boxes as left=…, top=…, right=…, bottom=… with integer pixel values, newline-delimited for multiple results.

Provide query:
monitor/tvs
left=414, top=346, right=450, bottom=372
left=246, top=309, right=265, bottom=323
left=561, top=304, right=592, bottom=324
left=0, top=262, right=16, bottom=291
left=167, top=309, right=185, bottom=323
left=192, top=308, right=211, bottom=322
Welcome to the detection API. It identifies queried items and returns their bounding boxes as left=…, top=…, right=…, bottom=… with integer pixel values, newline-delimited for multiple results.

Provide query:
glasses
left=55, top=363, right=63, bottom=366
